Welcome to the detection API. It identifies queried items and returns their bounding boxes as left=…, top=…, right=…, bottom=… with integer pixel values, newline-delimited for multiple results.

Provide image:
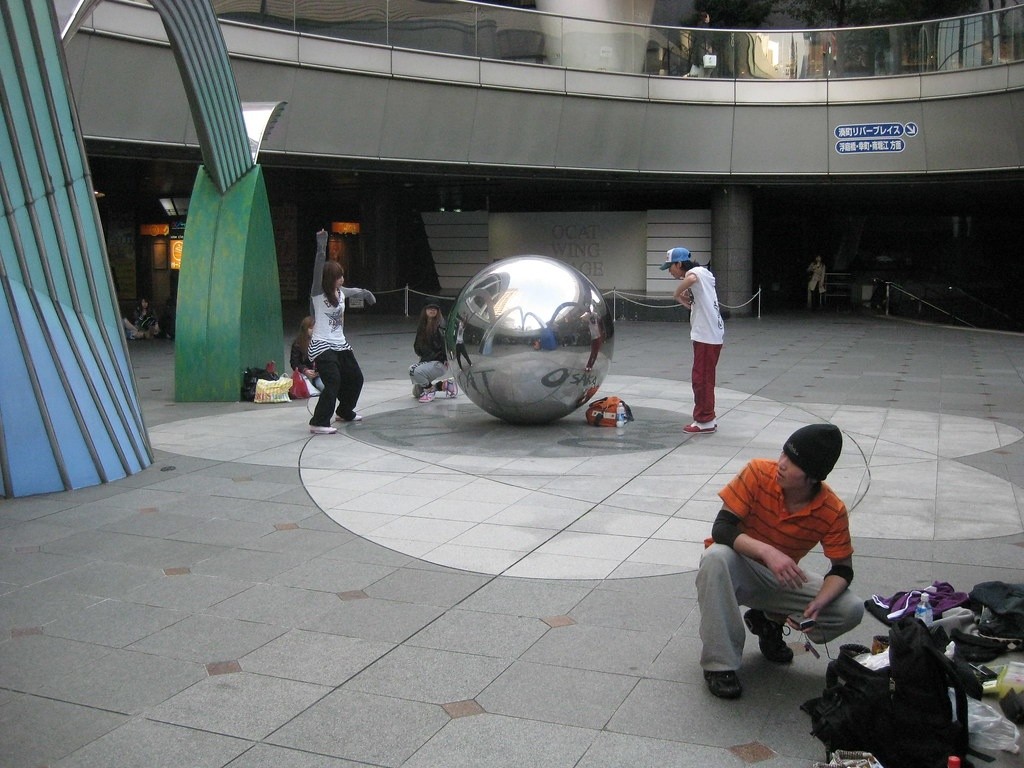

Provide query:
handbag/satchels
left=242, top=368, right=311, bottom=404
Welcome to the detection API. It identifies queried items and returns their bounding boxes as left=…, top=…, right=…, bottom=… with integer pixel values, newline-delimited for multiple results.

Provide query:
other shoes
left=471, top=364, right=473, bottom=366
left=460, top=369, right=462, bottom=371
left=146, top=327, right=154, bottom=339
left=586, top=367, right=592, bottom=370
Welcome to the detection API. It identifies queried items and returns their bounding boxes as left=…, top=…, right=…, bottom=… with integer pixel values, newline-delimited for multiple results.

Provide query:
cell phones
left=969, top=661, right=997, bottom=680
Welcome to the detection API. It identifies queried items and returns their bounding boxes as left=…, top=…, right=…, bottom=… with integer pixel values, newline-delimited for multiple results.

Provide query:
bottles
left=947, top=755, right=960, bottom=768
left=914, top=592, right=933, bottom=625
left=616, top=402, right=624, bottom=428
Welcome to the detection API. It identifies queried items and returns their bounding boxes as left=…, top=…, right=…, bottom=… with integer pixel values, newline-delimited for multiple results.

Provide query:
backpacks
left=586, top=396, right=634, bottom=427
left=799, top=617, right=995, bottom=768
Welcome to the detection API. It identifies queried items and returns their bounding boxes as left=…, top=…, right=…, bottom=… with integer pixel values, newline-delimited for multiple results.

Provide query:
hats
left=783, top=424, right=843, bottom=480
left=659, top=247, right=692, bottom=270
left=422, top=296, right=441, bottom=308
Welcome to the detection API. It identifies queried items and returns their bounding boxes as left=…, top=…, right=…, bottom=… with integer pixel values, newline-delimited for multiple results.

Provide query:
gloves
left=317, top=229, right=328, bottom=247
left=363, top=289, right=377, bottom=306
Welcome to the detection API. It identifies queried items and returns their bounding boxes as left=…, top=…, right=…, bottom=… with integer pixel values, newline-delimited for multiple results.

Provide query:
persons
left=694, top=424, right=865, bottom=699
left=408, top=296, right=459, bottom=403
left=683, top=11, right=717, bottom=78
left=659, top=247, right=724, bottom=434
left=123, top=295, right=161, bottom=340
left=579, top=302, right=605, bottom=372
left=306, top=228, right=377, bottom=434
left=806, top=254, right=826, bottom=313
left=454, top=311, right=473, bottom=372
left=290, top=313, right=326, bottom=391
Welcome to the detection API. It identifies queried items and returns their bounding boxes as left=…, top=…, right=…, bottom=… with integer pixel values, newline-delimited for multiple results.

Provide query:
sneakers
left=443, top=377, right=458, bottom=399
left=703, top=669, right=742, bottom=699
left=335, top=415, right=362, bottom=421
left=309, top=425, right=337, bottom=434
left=683, top=418, right=717, bottom=434
left=419, top=387, right=436, bottom=402
left=744, top=609, right=793, bottom=663
